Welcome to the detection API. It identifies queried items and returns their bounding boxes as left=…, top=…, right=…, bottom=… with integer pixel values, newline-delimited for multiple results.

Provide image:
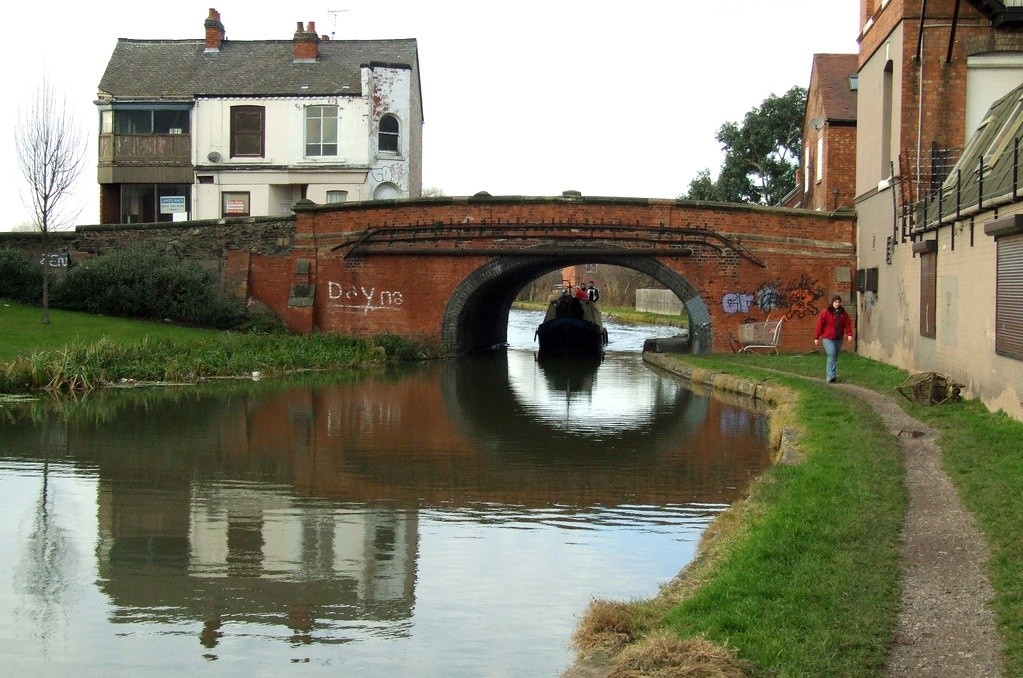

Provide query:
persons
left=575, top=283, right=588, bottom=300
left=586, top=280, right=599, bottom=303
left=814, top=294, right=852, bottom=383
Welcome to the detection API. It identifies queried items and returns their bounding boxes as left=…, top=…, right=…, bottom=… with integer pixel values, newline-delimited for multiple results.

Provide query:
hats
left=832, top=295, right=841, bottom=302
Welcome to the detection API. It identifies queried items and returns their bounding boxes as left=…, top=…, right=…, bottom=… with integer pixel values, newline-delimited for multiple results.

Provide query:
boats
left=537, top=280, right=609, bottom=352
left=537, top=353, right=599, bottom=428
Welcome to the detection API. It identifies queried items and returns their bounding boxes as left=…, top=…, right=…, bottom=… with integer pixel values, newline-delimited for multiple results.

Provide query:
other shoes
left=829, top=378, right=836, bottom=384
